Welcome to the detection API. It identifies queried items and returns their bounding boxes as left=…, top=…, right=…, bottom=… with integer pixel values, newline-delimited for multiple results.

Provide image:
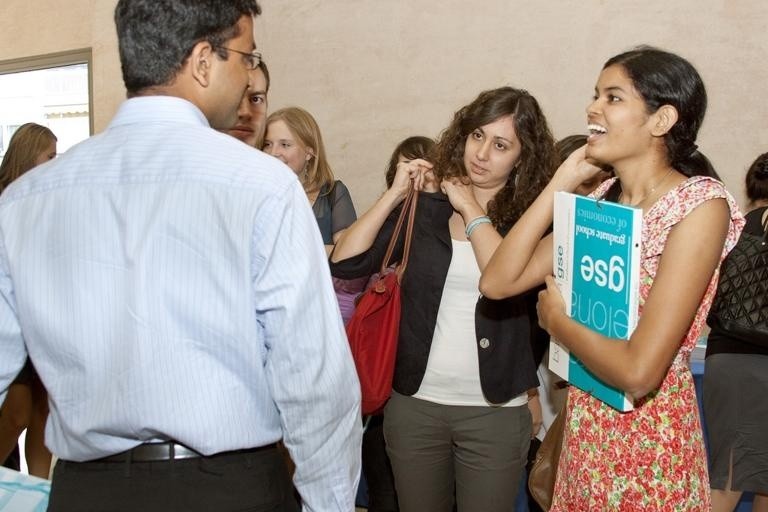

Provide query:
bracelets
left=465, top=215, right=493, bottom=241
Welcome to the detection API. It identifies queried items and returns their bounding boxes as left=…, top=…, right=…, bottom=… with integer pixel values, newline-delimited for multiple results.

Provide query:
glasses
left=210, top=43, right=260, bottom=71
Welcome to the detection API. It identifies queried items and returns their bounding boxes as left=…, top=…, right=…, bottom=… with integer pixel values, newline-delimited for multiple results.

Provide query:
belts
left=91, top=440, right=286, bottom=462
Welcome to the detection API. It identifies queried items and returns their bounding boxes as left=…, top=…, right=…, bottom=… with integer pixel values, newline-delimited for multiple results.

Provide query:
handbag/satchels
left=529, top=390, right=566, bottom=511
left=346, top=177, right=420, bottom=417
left=706, top=210, right=768, bottom=343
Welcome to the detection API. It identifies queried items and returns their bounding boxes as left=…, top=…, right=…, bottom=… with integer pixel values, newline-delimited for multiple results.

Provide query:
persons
left=387, top=137, right=436, bottom=188
left=703, top=152, right=768, bottom=511
left=0, top=0, right=363, bottom=512
left=225, top=62, right=270, bottom=147
left=477, top=44, right=746, bottom=511
left=554, top=134, right=613, bottom=201
left=328, top=86, right=563, bottom=512
left=1, top=122, right=57, bottom=480
left=264, top=105, right=357, bottom=259
